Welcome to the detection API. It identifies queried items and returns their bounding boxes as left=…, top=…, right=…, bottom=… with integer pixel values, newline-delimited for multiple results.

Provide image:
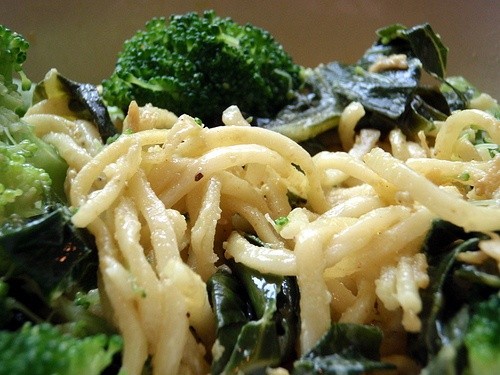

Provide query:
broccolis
left=0, top=1, right=500, bottom=375
